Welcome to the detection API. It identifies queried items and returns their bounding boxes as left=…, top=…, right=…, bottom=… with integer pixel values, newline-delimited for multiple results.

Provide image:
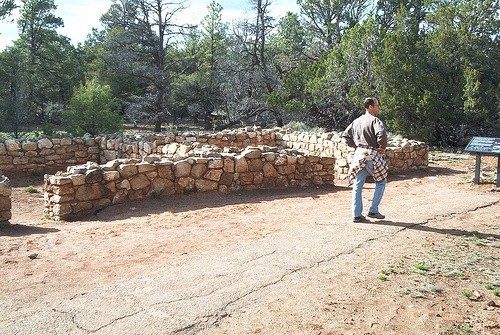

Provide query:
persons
left=341, top=97, right=389, bottom=222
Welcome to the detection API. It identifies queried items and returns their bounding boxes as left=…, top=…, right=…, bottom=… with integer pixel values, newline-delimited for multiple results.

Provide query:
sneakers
left=368, top=210, right=385, bottom=219
left=354, top=215, right=366, bottom=223
